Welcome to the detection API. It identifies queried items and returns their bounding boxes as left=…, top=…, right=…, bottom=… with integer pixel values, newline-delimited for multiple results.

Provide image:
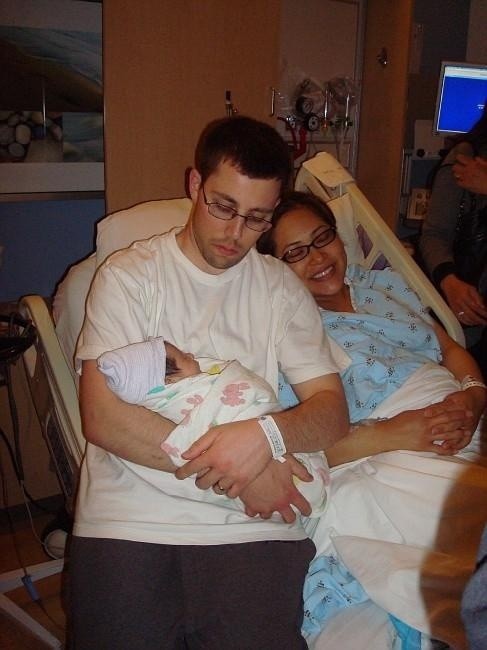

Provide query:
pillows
left=96, top=193, right=366, bottom=276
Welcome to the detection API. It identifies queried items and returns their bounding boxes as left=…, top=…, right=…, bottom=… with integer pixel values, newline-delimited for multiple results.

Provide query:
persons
left=97, top=336, right=330, bottom=515
left=417, top=103, right=487, bottom=373
left=61, top=114, right=351, bottom=649
left=256, top=191, right=487, bottom=556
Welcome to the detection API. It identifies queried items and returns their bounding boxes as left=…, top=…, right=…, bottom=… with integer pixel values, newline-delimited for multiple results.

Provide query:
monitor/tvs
left=433, top=59, right=487, bottom=138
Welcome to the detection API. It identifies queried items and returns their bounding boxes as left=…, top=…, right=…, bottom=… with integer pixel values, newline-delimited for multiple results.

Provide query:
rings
left=216, top=483, right=227, bottom=493
left=459, top=312, right=464, bottom=316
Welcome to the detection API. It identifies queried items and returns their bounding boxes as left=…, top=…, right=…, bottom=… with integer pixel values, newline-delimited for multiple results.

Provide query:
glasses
left=272, top=224, right=340, bottom=266
left=198, top=174, right=277, bottom=236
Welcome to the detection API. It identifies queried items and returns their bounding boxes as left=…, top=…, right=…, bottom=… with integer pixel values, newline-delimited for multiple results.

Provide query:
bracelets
left=460, top=373, right=487, bottom=391
left=259, top=413, right=288, bottom=466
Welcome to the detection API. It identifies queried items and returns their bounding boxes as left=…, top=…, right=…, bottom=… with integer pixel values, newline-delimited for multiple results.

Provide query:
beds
left=17, top=152, right=467, bottom=650
